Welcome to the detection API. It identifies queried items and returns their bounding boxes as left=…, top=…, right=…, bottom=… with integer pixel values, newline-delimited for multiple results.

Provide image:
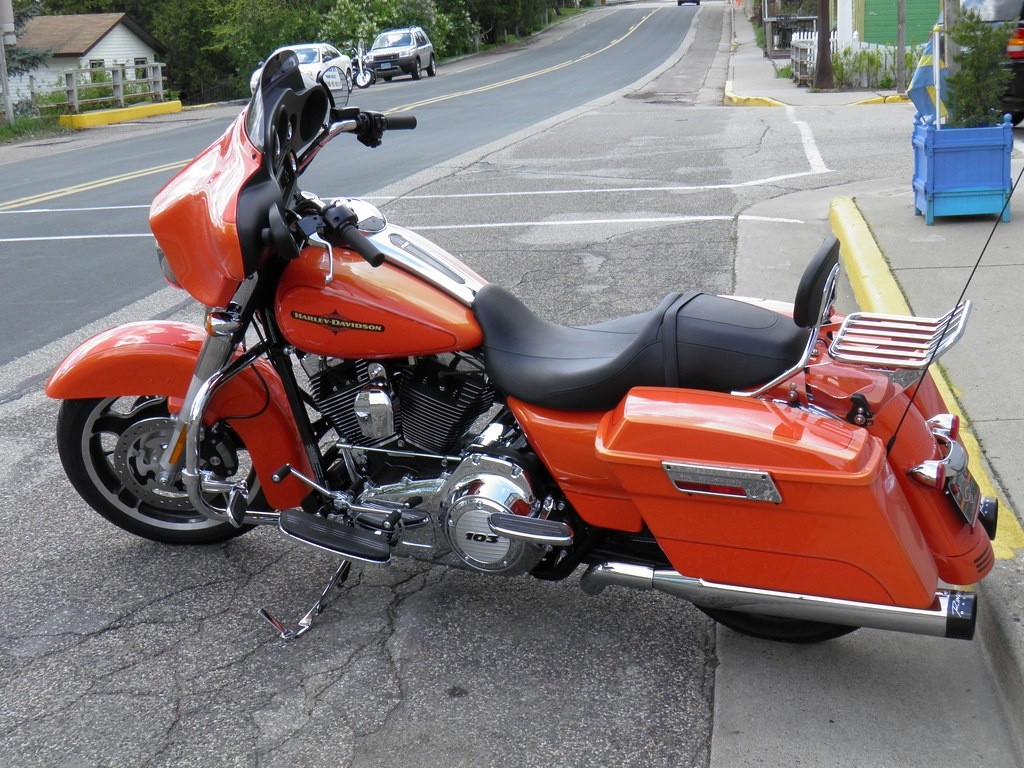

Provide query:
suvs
left=366, top=25, right=437, bottom=82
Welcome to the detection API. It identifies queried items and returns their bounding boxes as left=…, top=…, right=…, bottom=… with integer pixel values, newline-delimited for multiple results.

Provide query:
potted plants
left=911, top=2, right=1021, bottom=226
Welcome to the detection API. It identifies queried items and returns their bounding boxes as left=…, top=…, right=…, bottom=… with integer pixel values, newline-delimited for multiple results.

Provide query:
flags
left=906, top=22, right=952, bottom=126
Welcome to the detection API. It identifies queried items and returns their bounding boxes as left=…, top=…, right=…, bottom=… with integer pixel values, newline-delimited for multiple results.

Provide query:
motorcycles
left=46, top=48, right=999, bottom=644
left=345, top=38, right=375, bottom=90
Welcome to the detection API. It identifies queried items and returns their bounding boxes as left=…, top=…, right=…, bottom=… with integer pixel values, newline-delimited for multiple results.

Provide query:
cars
left=249, top=43, right=354, bottom=100
